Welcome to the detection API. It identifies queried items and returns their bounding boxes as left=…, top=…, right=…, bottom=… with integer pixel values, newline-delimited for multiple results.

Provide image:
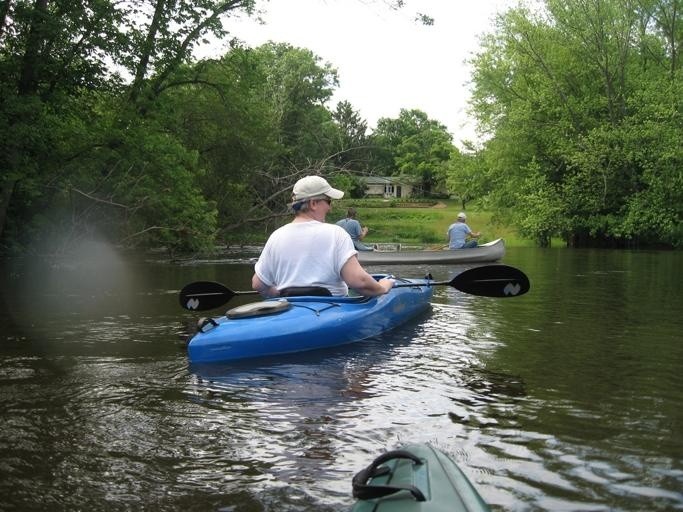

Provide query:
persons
left=446, top=212, right=482, bottom=249
left=335, top=207, right=369, bottom=251
left=251, top=175, right=397, bottom=298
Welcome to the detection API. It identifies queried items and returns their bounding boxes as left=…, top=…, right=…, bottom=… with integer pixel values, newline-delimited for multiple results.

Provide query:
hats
left=456, top=213, right=467, bottom=219
left=291, top=174, right=345, bottom=201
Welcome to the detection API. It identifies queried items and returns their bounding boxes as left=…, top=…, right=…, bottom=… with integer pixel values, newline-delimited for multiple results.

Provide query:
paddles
left=179, top=265, right=530, bottom=311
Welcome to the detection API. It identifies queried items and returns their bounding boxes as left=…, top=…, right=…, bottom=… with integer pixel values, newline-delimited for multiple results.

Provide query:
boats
left=350, top=442, right=491, bottom=510
left=188, top=274, right=434, bottom=364
left=354, top=238, right=504, bottom=263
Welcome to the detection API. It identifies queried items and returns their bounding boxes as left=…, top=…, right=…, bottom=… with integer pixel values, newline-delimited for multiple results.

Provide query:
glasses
left=313, top=199, right=331, bottom=205
